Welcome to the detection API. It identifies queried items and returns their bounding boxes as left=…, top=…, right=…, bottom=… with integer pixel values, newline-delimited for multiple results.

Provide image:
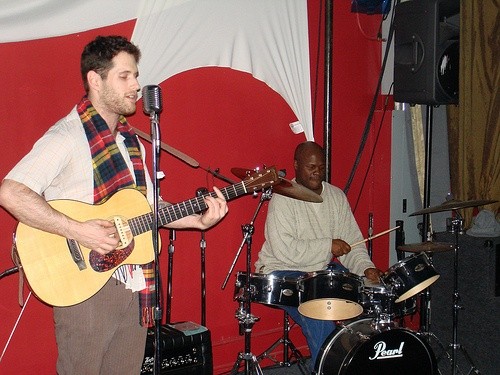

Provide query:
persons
left=254, top=141, right=383, bottom=370
left=1, top=35, right=228, bottom=375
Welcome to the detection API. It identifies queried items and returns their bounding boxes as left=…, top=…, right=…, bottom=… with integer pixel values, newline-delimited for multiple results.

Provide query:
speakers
left=393, top=0, right=461, bottom=107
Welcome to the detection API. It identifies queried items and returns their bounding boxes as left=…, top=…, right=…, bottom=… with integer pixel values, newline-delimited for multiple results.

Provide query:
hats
left=466, top=210, right=500, bottom=237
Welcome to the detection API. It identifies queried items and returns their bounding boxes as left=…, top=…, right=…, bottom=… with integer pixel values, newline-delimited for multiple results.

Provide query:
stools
left=256, top=304, right=309, bottom=375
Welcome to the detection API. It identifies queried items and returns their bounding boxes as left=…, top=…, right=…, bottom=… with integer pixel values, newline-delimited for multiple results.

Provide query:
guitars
left=4, top=166, right=283, bottom=307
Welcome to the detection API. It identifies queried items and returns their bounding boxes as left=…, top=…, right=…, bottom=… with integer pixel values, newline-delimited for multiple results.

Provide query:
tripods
left=415, top=209, right=481, bottom=375
left=222, top=187, right=274, bottom=375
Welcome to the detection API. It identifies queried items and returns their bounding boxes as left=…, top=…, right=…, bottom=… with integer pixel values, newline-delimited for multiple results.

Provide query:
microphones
left=142, top=85, right=163, bottom=159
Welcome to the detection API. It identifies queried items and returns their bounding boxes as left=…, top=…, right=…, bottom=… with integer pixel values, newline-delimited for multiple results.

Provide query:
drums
left=297, top=269, right=364, bottom=320
left=393, top=294, right=417, bottom=318
left=381, top=253, right=442, bottom=302
left=234, top=270, right=308, bottom=304
left=314, top=318, right=441, bottom=375
left=360, top=285, right=401, bottom=320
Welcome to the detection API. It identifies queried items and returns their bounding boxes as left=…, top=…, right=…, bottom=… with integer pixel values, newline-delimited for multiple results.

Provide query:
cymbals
left=396, top=241, right=459, bottom=252
left=407, top=193, right=500, bottom=217
left=231, top=166, right=324, bottom=204
